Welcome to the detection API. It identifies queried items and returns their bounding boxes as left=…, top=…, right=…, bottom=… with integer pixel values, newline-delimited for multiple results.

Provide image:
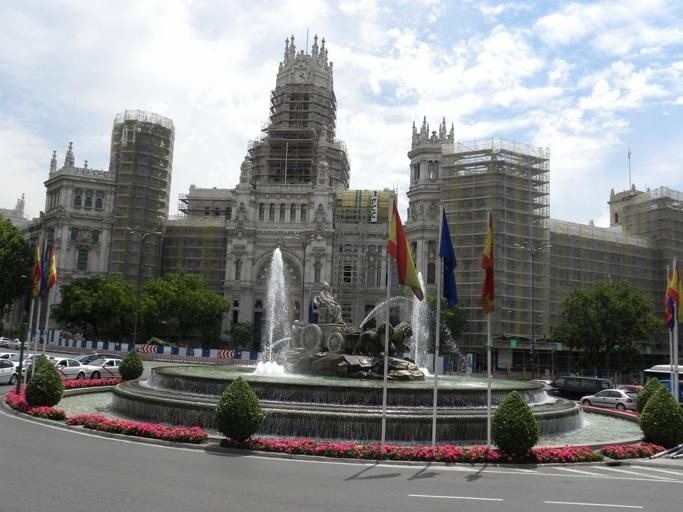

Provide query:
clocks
left=295, top=71, right=308, bottom=83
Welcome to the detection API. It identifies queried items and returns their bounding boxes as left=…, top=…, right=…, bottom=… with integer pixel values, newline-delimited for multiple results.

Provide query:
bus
left=642, top=363, right=683, bottom=406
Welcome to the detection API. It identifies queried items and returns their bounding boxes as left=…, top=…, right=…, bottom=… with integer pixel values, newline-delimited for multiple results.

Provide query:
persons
left=320, top=282, right=343, bottom=323
left=328, top=286, right=337, bottom=303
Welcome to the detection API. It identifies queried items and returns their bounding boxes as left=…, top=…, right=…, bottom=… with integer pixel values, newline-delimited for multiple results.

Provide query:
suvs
left=549, top=374, right=613, bottom=402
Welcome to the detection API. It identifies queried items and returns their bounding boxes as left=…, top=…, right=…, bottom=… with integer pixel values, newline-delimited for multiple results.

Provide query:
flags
left=289, top=292, right=296, bottom=321
left=437, top=206, right=458, bottom=310
left=32, top=240, right=41, bottom=298
left=46, top=247, right=56, bottom=290
left=479, top=211, right=495, bottom=314
left=38, top=246, right=47, bottom=295
left=664, top=274, right=675, bottom=331
left=386, top=198, right=424, bottom=303
left=667, top=263, right=683, bottom=330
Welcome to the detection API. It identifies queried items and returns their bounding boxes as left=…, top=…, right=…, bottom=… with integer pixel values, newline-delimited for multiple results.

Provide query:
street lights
left=513, top=238, right=553, bottom=380
left=284, top=231, right=325, bottom=323
left=123, top=224, right=165, bottom=344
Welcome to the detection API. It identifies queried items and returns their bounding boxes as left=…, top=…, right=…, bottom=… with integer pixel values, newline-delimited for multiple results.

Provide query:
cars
left=580, top=388, right=639, bottom=412
left=530, top=378, right=553, bottom=395
left=615, top=384, right=644, bottom=396
left=0, top=335, right=124, bottom=387
